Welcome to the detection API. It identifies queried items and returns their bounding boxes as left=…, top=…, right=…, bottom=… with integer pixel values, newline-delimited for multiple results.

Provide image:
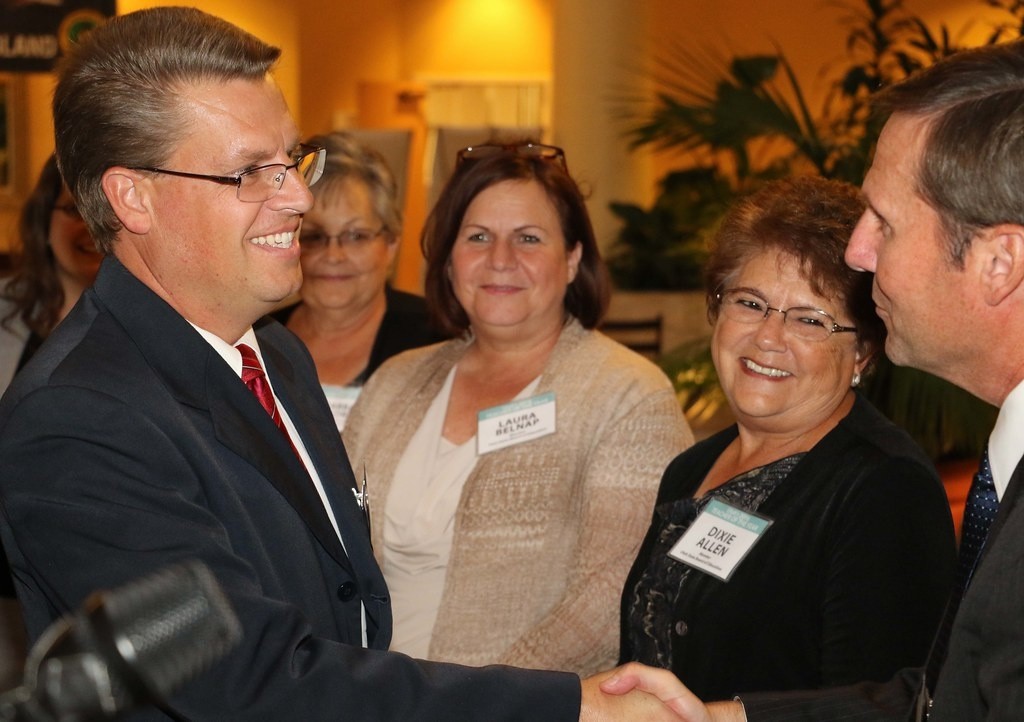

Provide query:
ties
left=238, top=343, right=310, bottom=473
left=926, top=445, right=998, bottom=667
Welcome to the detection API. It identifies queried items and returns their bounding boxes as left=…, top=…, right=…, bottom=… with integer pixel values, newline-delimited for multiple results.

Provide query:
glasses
left=132, top=147, right=327, bottom=201
left=716, top=288, right=862, bottom=341
left=298, top=216, right=387, bottom=248
left=54, top=202, right=79, bottom=215
left=457, top=141, right=565, bottom=166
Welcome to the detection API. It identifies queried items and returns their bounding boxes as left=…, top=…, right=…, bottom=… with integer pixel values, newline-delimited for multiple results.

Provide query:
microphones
left=1, top=557, right=242, bottom=722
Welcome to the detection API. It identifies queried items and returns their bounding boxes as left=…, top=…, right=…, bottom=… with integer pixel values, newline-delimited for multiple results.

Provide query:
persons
left=0, top=7, right=681, bottom=722
left=0, top=150, right=102, bottom=396
left=619, top=174, right=958, bottom=701
left=269, top=129, right=458, bottom=434
left=340, top=151, right=693, bottom=681
left=600, top=38, right=1024, bottom=722
